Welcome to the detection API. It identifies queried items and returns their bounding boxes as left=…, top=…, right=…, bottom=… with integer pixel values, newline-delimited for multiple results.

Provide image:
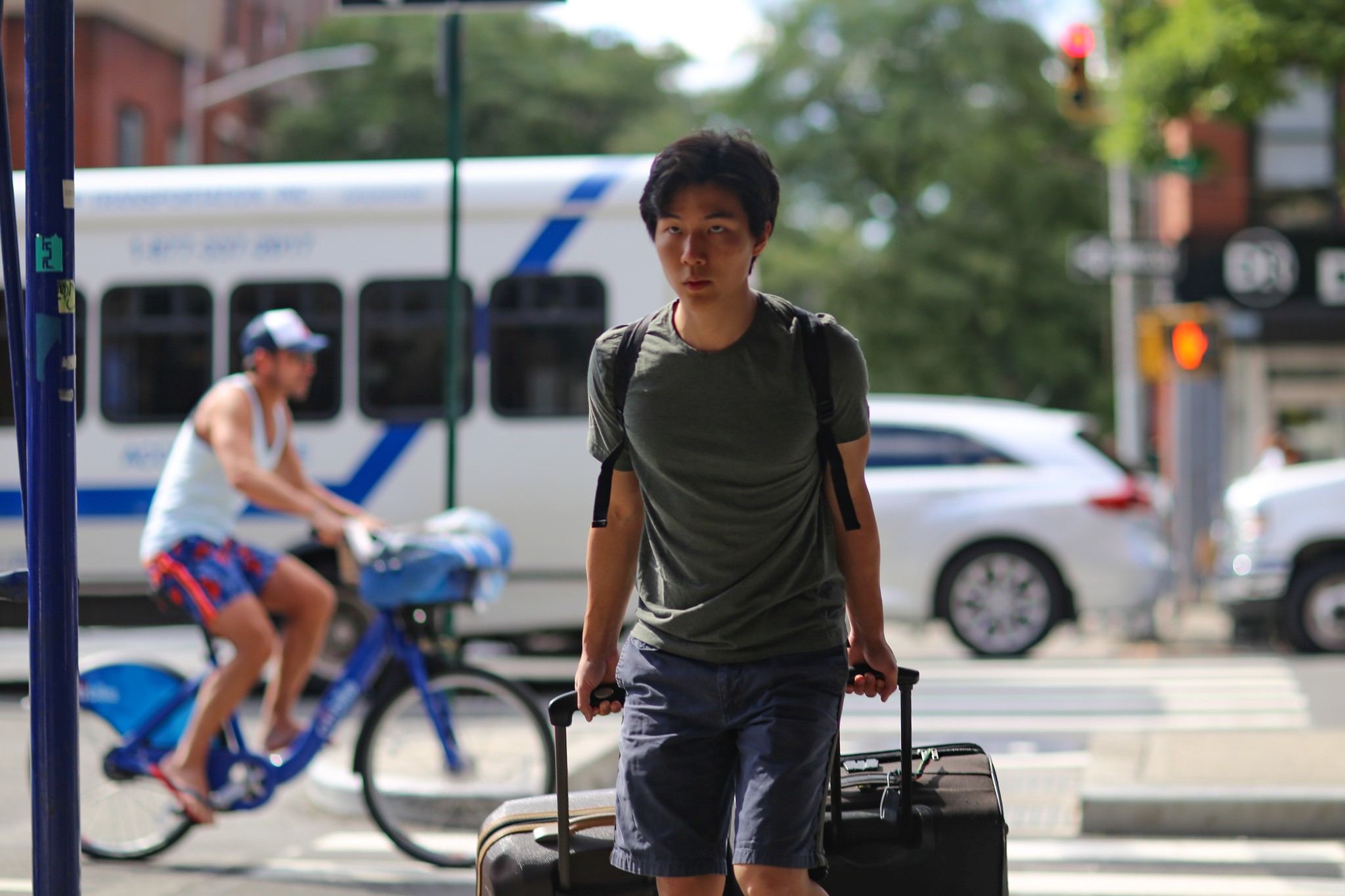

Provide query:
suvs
left=861, top=394, right=1175, bottom=657
left=1215, top=456, right=1345, bottom=659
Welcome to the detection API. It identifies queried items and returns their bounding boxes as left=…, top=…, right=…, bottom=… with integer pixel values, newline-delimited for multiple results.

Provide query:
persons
left=139, top=309, right=387, bottom=825
left=576, top=130, right=898, bottom=895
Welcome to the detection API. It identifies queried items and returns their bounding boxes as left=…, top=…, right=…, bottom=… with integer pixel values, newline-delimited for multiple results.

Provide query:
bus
left=1, top=150, right=761, bottom=690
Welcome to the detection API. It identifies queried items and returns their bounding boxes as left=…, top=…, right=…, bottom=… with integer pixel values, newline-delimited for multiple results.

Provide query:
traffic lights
left=1058, top=21, right=1098, bottom=126
left=1136, top=303, right=1221, bottom=380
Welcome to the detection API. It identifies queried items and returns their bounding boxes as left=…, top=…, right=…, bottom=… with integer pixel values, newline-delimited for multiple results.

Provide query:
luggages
left=808, top=667, right=1011, bottom=896
left=475, top=683, right=660, bottom=896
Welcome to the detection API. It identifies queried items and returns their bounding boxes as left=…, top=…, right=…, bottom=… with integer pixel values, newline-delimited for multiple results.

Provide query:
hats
left=241, top=307, right=329, bottom=358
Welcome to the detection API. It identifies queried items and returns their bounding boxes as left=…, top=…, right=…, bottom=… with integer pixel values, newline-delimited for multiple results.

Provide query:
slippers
left=147, top=762, right=216, bottom=825
left=260, top=726, right=330, bottom=757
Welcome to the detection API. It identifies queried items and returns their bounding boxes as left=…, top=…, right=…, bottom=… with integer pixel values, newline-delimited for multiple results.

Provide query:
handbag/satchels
left=358, top=506, right=512, bottom=605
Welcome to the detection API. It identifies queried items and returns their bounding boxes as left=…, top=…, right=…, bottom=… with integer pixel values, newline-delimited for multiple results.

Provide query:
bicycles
left=80, top=505, right=565, bottom=869
